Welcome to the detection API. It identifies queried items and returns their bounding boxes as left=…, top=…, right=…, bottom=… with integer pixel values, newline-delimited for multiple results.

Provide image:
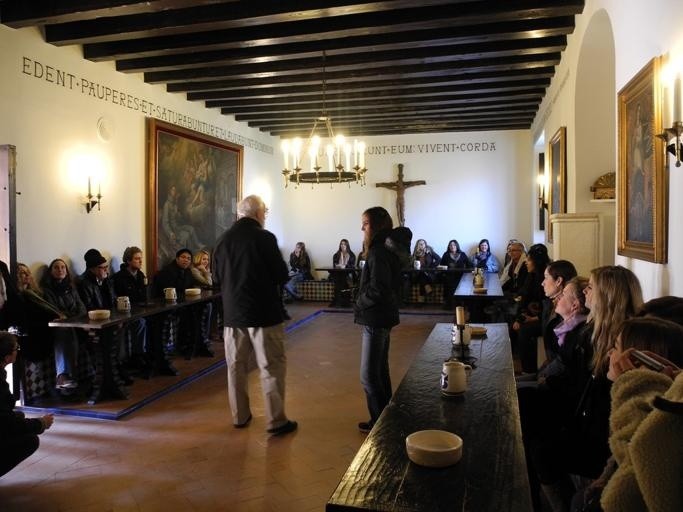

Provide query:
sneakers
left=198, top=342, right=214, bottom=358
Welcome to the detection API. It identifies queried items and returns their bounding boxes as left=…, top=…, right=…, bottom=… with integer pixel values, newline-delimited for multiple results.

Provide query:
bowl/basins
left=184, top=288, right=201, bottom=295
left=88, top=309, right=112, bottom=320
left=404, top=431, right=465, bottom=465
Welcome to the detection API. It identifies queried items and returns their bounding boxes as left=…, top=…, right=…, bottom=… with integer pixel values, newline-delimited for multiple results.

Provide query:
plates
left=473, top=288, right=488, bottom=294
left=470, top=325, right=489, bottom=334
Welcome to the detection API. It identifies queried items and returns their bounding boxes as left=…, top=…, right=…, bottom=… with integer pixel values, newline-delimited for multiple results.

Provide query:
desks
left=315, top=266, right=492, bottom=308
left=48, top=288, right=222, bottom=405
left=454, top=272, right=504, bottom=323
left=326, top=323, right=534, bottom=512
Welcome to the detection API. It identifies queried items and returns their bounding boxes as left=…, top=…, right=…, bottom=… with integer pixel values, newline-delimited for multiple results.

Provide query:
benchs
left=287, top=280, right=446, bottom=305
left=19, top=311, right=225, bottom=404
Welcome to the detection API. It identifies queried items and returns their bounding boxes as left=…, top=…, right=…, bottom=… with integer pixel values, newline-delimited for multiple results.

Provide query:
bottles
left=474, top=248, right=481, bottom=274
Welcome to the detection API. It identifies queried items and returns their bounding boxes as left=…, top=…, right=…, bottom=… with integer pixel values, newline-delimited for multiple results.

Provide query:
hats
left=84, top=249, right=106, bottom=267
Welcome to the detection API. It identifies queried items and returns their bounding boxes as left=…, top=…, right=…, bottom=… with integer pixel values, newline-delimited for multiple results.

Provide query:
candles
left=455, top=305, right=465, bottom=326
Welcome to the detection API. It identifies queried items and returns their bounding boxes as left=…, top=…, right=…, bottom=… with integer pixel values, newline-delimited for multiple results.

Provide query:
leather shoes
left=121, top=351, right=181, bottom=385
left=55, top=374, right=77, bottom=390
left=267, top=420, right=296, bottom=432
left=358, top=422, right=369, bottom=433
left=233, top=413, right=251, bottom=428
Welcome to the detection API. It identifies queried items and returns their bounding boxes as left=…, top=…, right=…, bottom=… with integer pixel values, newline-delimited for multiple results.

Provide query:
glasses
left=14, top=344, right=20, bottom=351
left=96, top=265, right=108, bottom=269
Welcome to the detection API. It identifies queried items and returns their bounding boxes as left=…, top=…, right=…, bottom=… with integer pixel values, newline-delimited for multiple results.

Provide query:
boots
left=424, top=284, right=432, bottom=295
left=416, top=296, right=425, bottom=307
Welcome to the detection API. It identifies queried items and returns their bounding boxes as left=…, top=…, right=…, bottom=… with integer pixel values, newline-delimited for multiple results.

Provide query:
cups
left=162, top=287, right=177, bottom=300
left=439, top=360, right=471, bottom=396
left=358, top=260, right=364, bottom=268
left=413, top=260, right=420, bottom=268
left=116, top=296, right=130, bottom=312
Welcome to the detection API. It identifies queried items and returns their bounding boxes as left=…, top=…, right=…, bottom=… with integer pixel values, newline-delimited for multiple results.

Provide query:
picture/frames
left=548, top=127, right=567, bottom=244
left=144, top=117, right=244, bottom=284
left=615, top=56, right=670, bottom=264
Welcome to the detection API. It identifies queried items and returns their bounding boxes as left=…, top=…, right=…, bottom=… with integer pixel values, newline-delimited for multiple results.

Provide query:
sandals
left=400, top=297, right=408, bottom=306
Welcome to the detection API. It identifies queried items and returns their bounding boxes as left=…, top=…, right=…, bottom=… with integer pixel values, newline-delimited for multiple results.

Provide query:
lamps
left=281, top=51, right=371, bottom=189
left=85, top=177, right=103, bottom=214
left=656, top=62, right=683, bottom=167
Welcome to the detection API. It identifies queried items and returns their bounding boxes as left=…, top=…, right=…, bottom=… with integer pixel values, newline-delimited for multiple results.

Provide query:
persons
left=16, top=247, right=213, bottom=395
left=484, top=239, right=555, bottom=375
left=412, top=239, right=500, bottom=310
left=161, top=185, right=208, bottom=257
left=189, top=146, right=213, bottom=208
left=332, top=239, right=355, bottom=306
left=631, top=103, right=645, bottom=207
left=0, top=331, right=54, bottom=477
left=211, top=195, right=297, bottom=437
left=354, top=206, right=404, bottom=433
left=0, top=260, right=12, bottom=331
left=284, top=242, right=314, bottom=305
left=517, top=260, right=682, bottom=512
